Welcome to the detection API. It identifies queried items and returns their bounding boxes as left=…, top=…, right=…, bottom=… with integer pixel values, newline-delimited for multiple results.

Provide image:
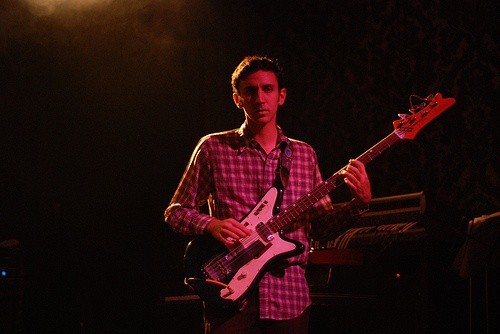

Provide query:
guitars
left=180, top=92, right=458, bottom=316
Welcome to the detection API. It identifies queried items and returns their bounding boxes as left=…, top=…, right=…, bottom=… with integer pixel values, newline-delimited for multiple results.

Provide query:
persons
left=164, top=55, right=371, bottom=334
